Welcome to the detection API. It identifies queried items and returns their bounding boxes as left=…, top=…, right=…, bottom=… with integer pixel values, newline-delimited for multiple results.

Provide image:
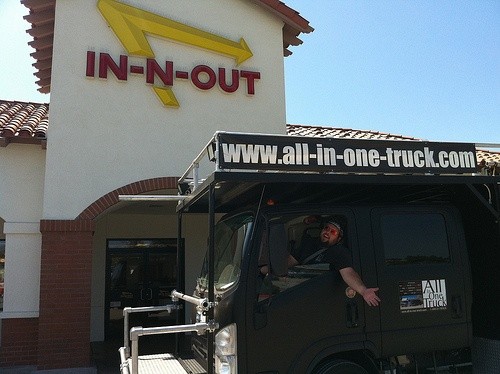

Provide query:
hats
left=328, top=217, right=345, bottom=237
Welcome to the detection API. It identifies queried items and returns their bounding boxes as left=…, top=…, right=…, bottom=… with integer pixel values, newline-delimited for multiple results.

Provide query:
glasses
left=324, top=225, right=338, bottom=236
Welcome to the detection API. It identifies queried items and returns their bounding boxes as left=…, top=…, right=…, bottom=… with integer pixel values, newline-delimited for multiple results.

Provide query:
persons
left=257, top=215, right=381, bottom=307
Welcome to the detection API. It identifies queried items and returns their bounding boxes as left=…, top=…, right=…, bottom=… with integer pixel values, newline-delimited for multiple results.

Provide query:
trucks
left=117, top=131, right=500, bottom=374
